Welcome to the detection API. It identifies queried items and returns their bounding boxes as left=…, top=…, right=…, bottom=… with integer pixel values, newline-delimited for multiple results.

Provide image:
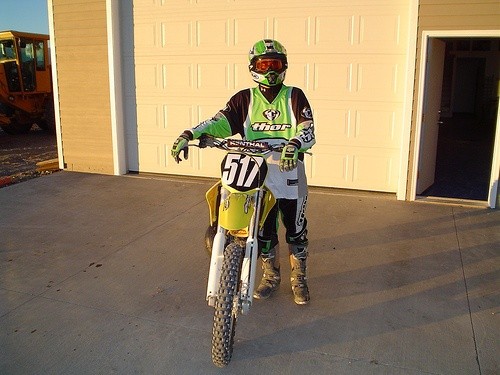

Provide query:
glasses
left=257, top=61, right=281, bottom=72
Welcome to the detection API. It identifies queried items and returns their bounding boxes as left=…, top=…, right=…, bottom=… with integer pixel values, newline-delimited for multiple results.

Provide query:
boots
left=290, top=254, right=311, bottom=305
left=253, top=253, right=281, bottom=299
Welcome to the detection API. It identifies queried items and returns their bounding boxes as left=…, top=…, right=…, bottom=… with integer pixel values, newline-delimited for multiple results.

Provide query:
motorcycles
left=188, top=132, right=312, bottom=368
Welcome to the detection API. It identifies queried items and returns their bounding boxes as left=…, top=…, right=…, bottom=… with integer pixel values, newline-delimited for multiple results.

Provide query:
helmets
left=248, top=39, right=288, bottom=87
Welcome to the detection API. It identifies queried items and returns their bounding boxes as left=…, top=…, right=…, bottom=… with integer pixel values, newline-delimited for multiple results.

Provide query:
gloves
left=170, top=130, right=193, bottom=164
left=279, top=139, right=301, bottom=173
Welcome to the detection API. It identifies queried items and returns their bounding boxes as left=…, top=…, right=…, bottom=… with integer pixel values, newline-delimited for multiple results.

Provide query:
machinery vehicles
left=0, top=29, right=56, bottom=140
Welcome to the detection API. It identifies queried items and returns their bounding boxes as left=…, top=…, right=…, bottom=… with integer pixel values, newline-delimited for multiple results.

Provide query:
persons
left=170, top=38, right=317, bottom=305
